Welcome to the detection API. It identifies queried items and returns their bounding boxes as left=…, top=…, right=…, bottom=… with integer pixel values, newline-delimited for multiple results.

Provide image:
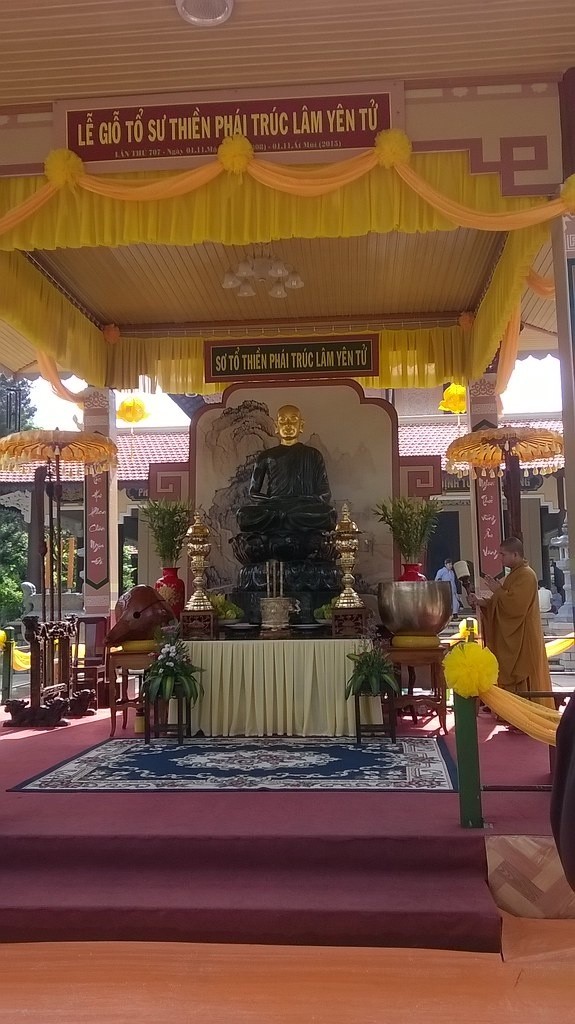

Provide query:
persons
left=538, top=579, right=558, bottom=614
left=238, top=405, right=338, bottom=531
left=433, top=560, right=460, bottom=620
left=467, top=537, right=557, bottom=733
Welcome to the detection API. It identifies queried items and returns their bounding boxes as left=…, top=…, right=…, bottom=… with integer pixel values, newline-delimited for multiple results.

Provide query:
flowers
left=139, top=626, right=205, bottom=710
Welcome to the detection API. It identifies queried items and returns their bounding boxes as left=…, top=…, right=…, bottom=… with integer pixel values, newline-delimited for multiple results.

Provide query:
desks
left=107, top=650, right=171, bottom=738
left=179, top=640, right=384, bottom=737
left=384, top=645, right=448, bottom=735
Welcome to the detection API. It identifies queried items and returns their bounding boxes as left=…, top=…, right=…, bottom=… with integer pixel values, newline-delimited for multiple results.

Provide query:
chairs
left=67, top=612, right=113, bottom=711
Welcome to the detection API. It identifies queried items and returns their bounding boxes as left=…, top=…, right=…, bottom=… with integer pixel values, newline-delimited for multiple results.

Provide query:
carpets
left=6, top=734, right=457, bottom=791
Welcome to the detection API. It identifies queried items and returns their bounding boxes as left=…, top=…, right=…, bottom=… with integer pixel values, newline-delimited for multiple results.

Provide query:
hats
left=444, top=559, right=452, bottom=564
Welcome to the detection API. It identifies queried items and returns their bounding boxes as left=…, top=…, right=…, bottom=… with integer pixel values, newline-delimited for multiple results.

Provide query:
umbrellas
left=442, top=424, right=564, bottom=480
left=0, top=427, right=119, bottom=484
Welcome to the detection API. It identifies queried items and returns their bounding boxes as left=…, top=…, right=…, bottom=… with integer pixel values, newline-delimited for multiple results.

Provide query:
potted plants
left=369, top=494, right=443, bottom=584
left=341, top=635, right=401, bottom=699
left=137, top=498, right=202, bottom=621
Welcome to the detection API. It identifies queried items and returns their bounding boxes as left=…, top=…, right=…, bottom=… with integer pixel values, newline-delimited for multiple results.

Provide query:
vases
left=161, top=682, right=180, bottom=695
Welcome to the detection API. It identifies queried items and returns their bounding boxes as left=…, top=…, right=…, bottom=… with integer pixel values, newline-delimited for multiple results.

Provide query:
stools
left=351, top=693, right=400, bottom=745
left=143, top=690, right=191, bottom=745
